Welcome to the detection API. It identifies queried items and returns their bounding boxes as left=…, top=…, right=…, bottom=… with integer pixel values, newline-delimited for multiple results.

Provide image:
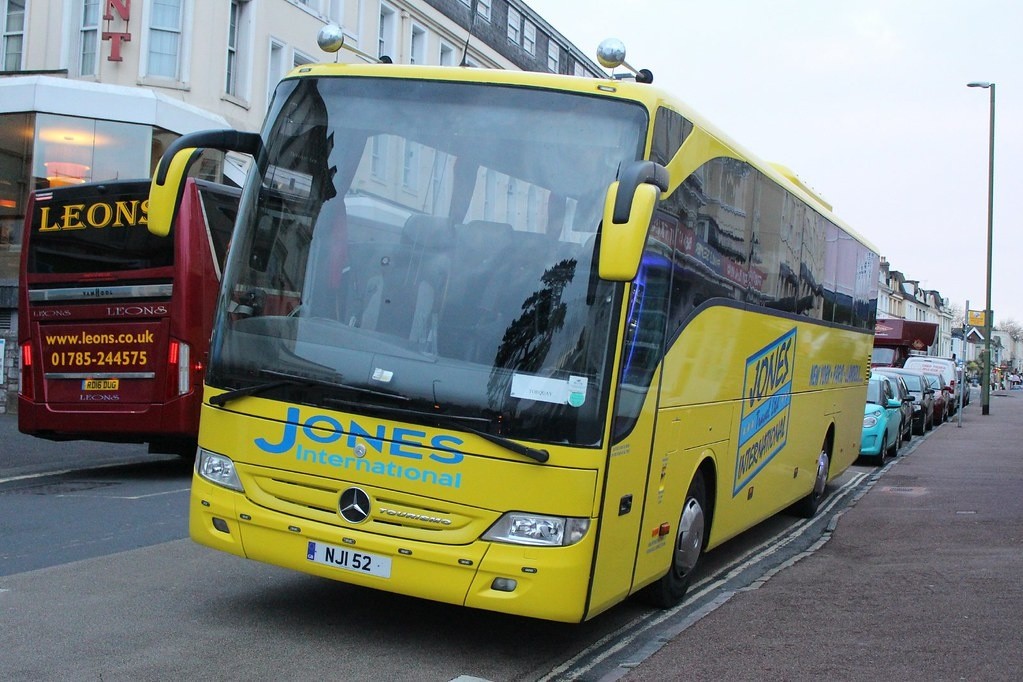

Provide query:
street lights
left=968, top=80, right=995, bottom=415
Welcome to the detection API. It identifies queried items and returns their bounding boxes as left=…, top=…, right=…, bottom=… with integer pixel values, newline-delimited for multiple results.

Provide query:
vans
left=902, top=357, right=959, bottom=417
left=869, top=317, right=939, bottom=370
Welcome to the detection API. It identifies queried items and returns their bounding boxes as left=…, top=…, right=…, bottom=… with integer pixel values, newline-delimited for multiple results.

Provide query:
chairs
left=382, top=214, right=853, bottom=383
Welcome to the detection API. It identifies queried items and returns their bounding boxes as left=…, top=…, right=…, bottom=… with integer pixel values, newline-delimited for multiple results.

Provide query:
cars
left=858, top=367, right=953, bottom=467
left=955, top=366, right=969, bottom=408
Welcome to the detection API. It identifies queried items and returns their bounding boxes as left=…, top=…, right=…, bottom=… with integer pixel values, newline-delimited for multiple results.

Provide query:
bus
left=144, top=24, right=883, bottom=624
left=16, top=177, right=246, bottom=456
